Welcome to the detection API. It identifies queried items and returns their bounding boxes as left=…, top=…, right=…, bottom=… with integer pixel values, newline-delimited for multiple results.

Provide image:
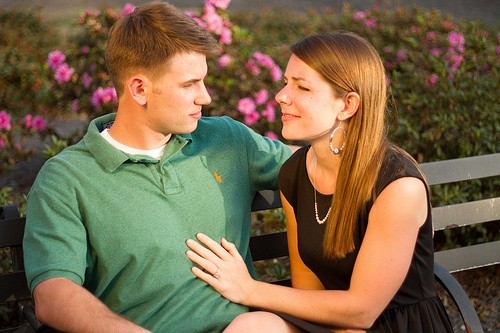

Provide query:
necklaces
left=314, top=158, right=332, bottom=224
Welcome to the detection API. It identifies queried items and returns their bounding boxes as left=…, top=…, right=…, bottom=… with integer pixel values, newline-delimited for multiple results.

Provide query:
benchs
left=0, top=150, right=500, bottom=333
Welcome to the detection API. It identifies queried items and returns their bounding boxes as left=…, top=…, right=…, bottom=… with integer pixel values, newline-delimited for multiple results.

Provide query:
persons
left=185, top=30, right=455, bottom=333
left=23, top=2, right=305, bottom=333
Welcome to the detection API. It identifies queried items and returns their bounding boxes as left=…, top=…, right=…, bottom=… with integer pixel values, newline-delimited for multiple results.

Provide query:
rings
left=214, top=269, right=223, bottom=279
